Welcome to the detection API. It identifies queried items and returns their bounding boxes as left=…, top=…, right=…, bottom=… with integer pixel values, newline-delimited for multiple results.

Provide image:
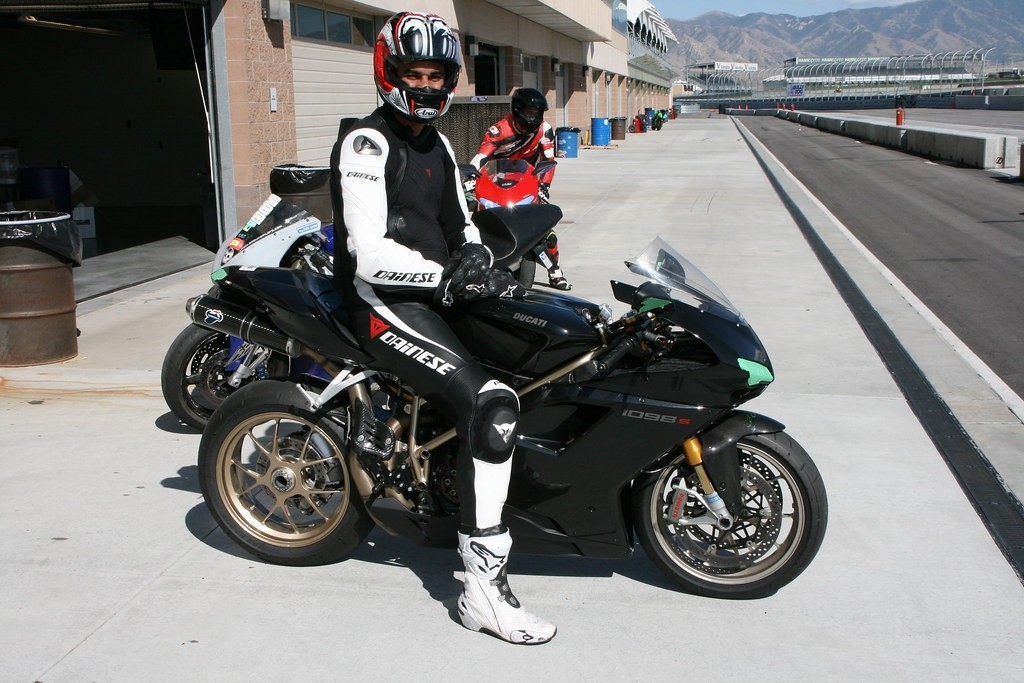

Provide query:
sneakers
left=549, top=270, right=571, bottom=291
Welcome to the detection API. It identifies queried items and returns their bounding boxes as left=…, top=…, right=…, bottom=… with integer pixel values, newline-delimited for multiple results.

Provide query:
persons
left=331, top=12, right=557, bottom=646
left=464, top=89, right=572, bottom=290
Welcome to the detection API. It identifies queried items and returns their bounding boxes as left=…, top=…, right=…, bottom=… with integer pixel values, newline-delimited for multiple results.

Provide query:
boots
left=453, top=528, right=556, bottom=644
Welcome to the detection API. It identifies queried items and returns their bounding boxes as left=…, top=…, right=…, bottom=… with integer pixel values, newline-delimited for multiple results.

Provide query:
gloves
left=434, top=242, right=490, bottom=306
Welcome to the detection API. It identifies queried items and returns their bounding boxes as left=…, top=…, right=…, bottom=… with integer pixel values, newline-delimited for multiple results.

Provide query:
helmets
left=512, top=89, right=548, bottom=130
left=374, top=12, right=462, bottom=122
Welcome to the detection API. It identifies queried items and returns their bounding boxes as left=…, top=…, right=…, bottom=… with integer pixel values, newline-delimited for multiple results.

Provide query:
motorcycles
left=652, top=109, right=668, bottom=131
left=183, top=237, right=829, bottom=603
left=458, top=155, right=557, bottom=288
left=160, top=190, right=337, bottom=433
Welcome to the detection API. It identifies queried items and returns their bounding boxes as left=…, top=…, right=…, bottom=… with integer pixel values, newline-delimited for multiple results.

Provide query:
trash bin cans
left=555, top=126, right=581, bottom=158
left=608, top=117, right=626, bottom=141
left=0, top=209, right=79, bottom=369
left=267, top=164, right=334, bottom=225
